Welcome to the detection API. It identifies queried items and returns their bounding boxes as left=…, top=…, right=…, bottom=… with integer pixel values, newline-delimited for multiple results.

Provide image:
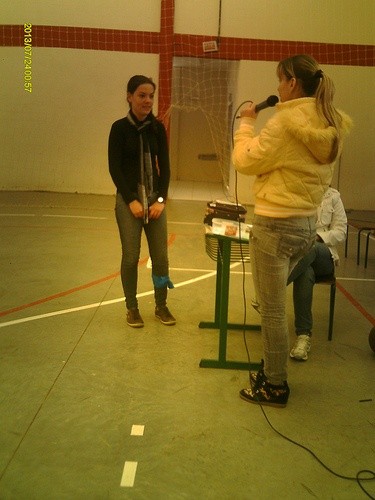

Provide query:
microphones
left=236, top=95, right=279, bottom=118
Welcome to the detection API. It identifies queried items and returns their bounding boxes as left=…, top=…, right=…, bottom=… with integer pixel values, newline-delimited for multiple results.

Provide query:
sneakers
left=289, top=335, right=311, bottom=360
left=251, top=298, right=260, bottom=311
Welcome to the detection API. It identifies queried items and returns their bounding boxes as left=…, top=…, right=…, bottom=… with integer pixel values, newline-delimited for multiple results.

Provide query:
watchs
left=155, top=196, right=166, bottom=204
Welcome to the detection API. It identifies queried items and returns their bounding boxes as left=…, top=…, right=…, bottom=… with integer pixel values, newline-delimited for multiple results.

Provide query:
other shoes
left=127, top=309, right=144, bottom=326
left=240, top=373, right=288, bottom=407
left=155, top=306, right=177, bottom=324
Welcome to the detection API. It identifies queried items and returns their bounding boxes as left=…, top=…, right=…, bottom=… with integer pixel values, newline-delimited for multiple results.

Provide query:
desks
left=198, top=220, right=263, bottom=371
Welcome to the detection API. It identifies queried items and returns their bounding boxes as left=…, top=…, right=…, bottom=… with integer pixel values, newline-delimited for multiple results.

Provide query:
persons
left=108, top=76, right=178, bottom=328
left=250, top=186, right=348, bottom=360
left=231, top=55, right=355, bottom=408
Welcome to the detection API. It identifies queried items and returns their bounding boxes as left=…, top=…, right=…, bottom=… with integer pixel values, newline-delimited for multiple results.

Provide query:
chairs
left=310, top=276, right=336, bottom=342
left=347, top=218, right=375, bottom=269
